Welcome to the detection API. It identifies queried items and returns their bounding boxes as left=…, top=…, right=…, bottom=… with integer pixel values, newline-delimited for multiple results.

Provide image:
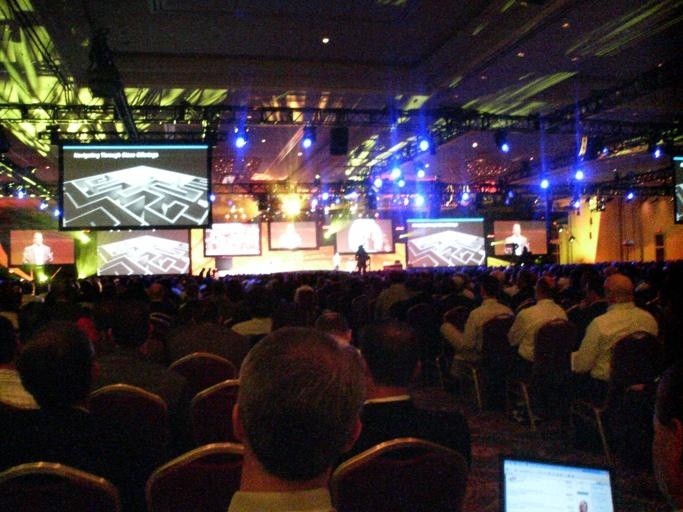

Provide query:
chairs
left=165, top=351, right=237, bottom=411
left=143, top=442, right=246, bottom=512
left=335, top=267, right=682, bottom=468
left=329, top=436, right=469, bottom=511
left=150, top=312, right=175, bottom=338
left=189, top=377, right=241, bottom=444
left=1, top=461, right=121, bottom=511
left=84, top=384, right=171, bottom=512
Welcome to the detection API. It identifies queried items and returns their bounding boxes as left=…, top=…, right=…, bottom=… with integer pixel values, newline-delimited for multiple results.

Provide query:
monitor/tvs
left=497, top=452, right=617, bottom=511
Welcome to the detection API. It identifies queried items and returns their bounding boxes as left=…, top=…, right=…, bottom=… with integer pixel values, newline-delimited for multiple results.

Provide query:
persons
left=355, top=245, right=371, bottom=272
left=21, top=231, right=54, bottom=263
left=0, top=260, right=683, bottom=512
left=504, top=223, right=531, bottom=256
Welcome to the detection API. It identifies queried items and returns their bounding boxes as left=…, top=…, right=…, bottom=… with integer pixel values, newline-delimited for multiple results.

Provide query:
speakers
left=655, top=248, right=665, bottom=262
left=654, top=234, right=664, bottom=246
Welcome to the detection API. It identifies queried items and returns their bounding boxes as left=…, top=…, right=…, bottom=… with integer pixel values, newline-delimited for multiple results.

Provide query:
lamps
left=417, top=134, right=436, bottom=155
left=303, top=124, right=315, bottom=148
left=230, top=125, right=253, bottom=148
